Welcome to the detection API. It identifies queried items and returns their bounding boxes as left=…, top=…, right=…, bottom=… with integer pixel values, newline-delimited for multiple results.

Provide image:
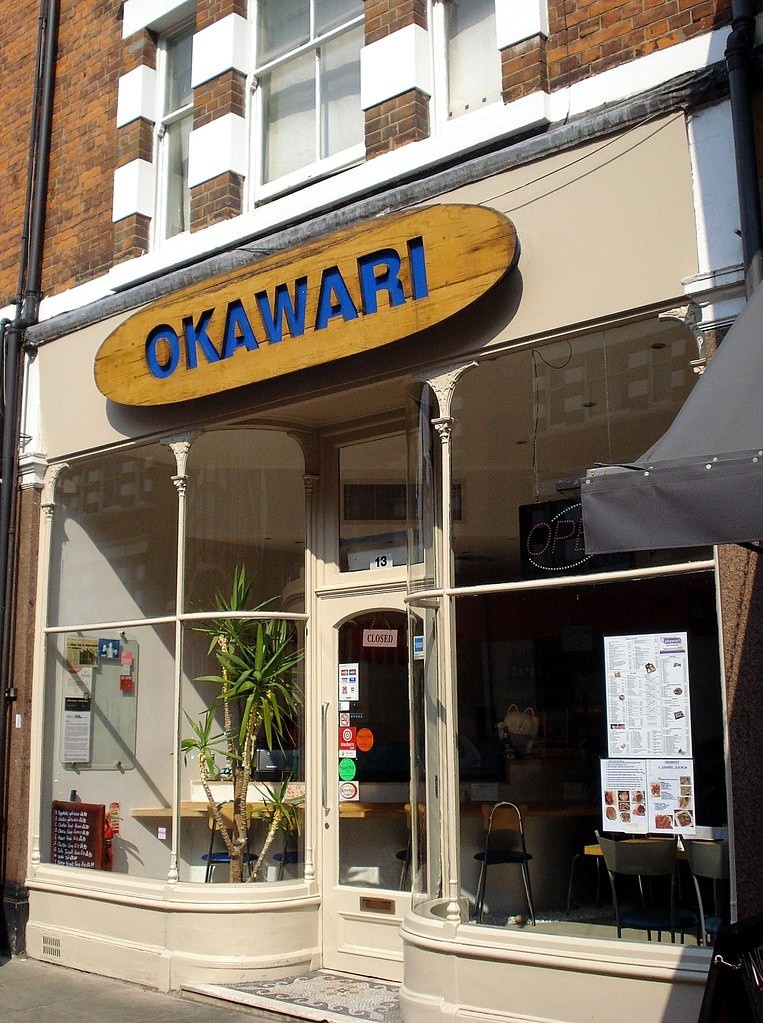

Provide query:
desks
left=586, top=836, right=729, bottom=857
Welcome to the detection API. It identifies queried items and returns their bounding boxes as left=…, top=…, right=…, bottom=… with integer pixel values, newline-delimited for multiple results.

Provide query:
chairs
left=199, top=800, right=259, bottom=883
left=679, top=833, right=731, bottom=946
left=396, top=804, right=426, bottom=891
left=590, top=830, right=701, bottom=945
left=474, top=800, right=536, bottom=925
left=272, top=800, right=305, bottom=880
left=566, top=822, right=646, bottom=920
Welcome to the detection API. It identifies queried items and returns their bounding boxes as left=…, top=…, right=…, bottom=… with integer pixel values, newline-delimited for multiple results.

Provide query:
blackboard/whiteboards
left=50, top=800, right=105, bottom=871
